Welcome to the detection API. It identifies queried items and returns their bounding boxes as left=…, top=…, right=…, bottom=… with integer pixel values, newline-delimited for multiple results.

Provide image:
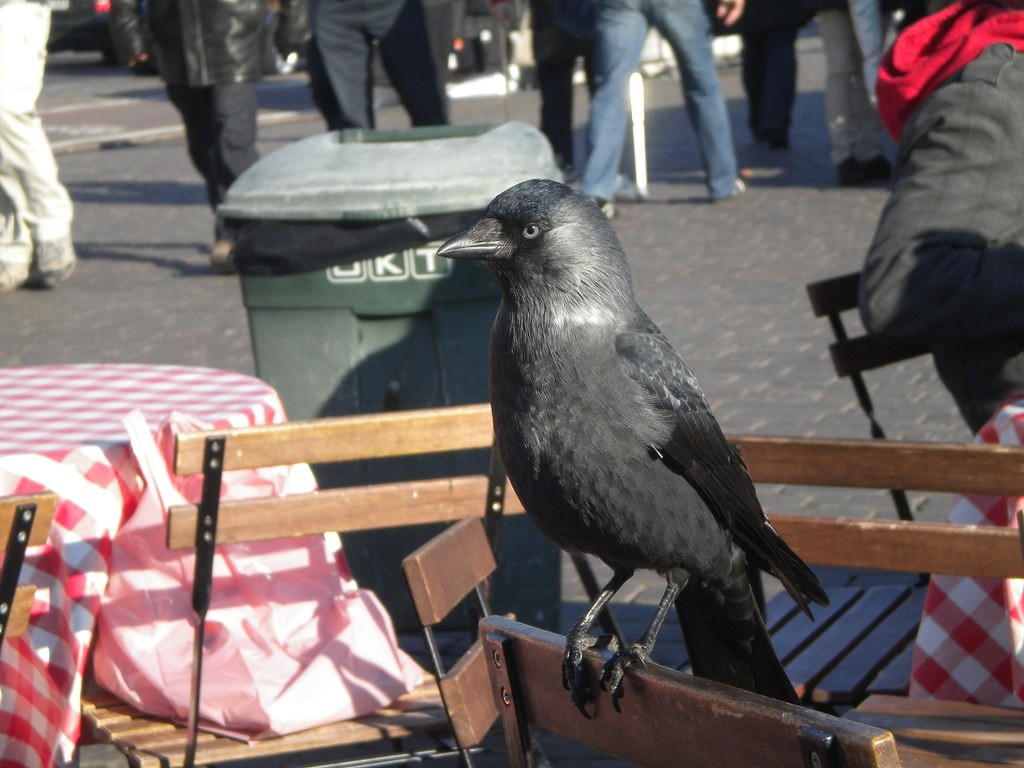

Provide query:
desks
left=905, top=389, right=1024, bottom=710
left=0, top=362, right=435, bottom=768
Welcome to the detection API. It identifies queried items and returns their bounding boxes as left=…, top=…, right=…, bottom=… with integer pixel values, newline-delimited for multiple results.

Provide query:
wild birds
left=435, top=179, right=829, bottom=721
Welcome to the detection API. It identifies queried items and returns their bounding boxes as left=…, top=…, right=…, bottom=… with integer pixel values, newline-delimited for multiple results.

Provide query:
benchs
left=80, top=402, right=525, bottom=768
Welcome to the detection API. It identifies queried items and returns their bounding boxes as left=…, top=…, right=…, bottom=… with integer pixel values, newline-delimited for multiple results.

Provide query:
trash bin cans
left=213, top=120, right=568, bottom=637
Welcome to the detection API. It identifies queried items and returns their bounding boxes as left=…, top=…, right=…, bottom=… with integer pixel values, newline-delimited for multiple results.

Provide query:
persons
left=0, top=0, right=896, bottom=292
left=858, top=0, right=1024, bottom=440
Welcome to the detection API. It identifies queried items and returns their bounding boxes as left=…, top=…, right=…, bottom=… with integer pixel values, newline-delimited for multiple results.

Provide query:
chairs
left=403, top=515, right=516, bottom=768
left=479, top=615, right=1024, bottom=768
left=0, top=490, right=58, bottom=656
left=806, top=272, right=930, bottom=520
left=679, top=565, right=930, bottom=705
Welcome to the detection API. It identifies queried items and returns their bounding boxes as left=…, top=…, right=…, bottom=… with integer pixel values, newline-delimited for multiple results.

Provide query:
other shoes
left=710, top=176, right=745, bottom=205
left=593, top=196, right=616, bottom=220
left=211, top=238, right=232, bottom=266
left=839, top=153, right=894, bottom=186
